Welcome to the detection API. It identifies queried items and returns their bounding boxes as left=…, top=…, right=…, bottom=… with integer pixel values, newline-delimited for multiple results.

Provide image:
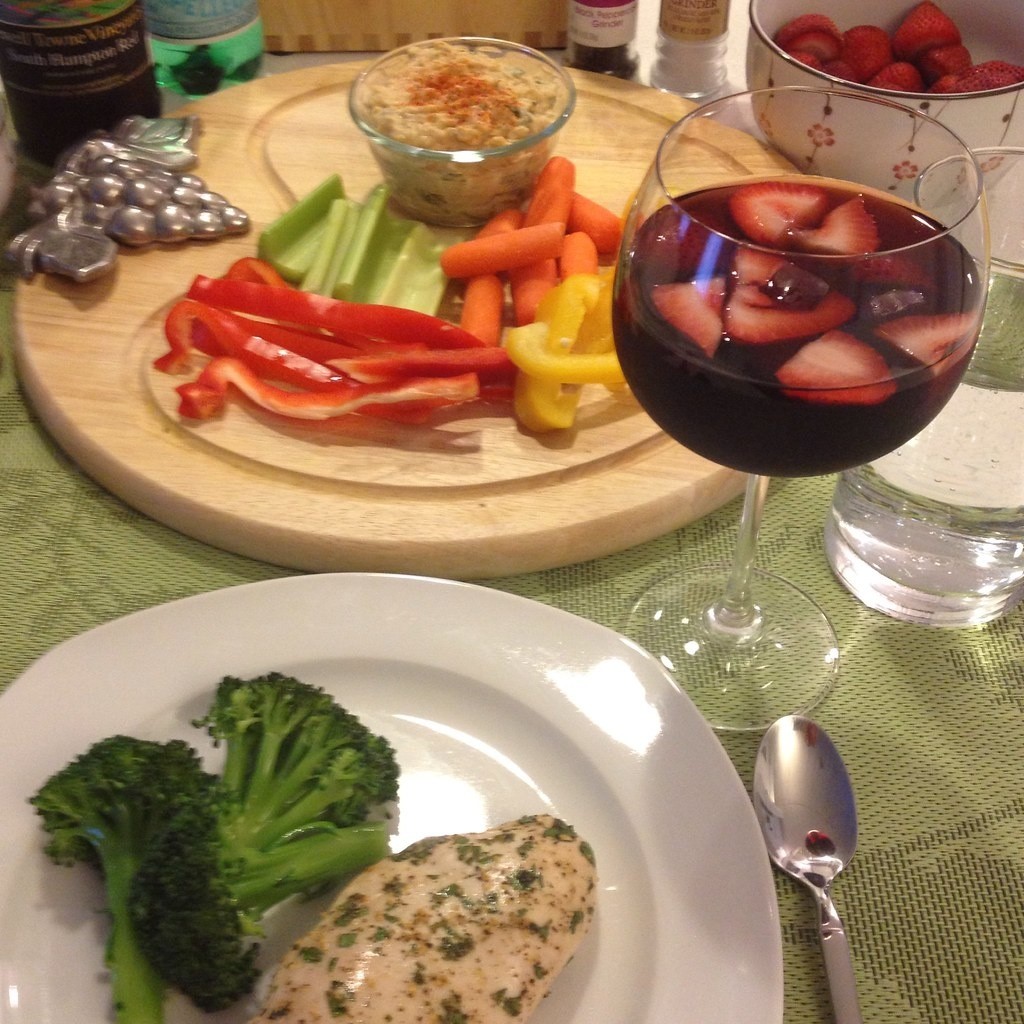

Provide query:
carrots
left=437, top=153, right=626, bottom=343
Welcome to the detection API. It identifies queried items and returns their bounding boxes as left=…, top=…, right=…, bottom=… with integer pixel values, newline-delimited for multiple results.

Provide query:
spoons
left=751, top=716, right=862, bottom=1024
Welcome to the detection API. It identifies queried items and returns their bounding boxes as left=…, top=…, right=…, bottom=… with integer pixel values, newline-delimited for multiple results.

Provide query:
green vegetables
left=261, top=814, right=596, bottom=1024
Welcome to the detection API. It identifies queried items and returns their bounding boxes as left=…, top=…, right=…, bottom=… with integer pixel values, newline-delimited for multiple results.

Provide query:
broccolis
left=25, top=669, right=400, bottom=1024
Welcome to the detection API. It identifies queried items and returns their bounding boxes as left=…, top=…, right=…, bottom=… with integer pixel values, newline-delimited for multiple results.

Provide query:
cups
left=822, top=145, right=1024, bottom=626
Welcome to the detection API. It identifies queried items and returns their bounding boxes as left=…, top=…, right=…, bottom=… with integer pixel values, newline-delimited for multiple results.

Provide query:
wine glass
left=610, top=82, right=993, bottom=730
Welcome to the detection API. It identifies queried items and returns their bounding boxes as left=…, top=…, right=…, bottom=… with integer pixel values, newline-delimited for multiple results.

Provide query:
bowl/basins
left=348, top=36, right=576, bottom=229
left=745, top=1, right=1024, bottom=207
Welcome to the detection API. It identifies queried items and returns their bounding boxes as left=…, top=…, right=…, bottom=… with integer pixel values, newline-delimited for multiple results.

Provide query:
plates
left=0, top=570, right=784, bottom=1024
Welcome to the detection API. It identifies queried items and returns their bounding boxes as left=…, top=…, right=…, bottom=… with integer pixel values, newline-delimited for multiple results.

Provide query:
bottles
left=0, top=0, right=162, bottom=168
left=649, top=0, right=731, bottom=99
left=566, top=0, right=642, bottom=80
left=144, top=0, right=266, bottom=98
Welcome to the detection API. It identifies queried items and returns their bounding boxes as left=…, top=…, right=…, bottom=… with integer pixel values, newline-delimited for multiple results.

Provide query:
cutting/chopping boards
left=13, top=56, right=803, bottom=573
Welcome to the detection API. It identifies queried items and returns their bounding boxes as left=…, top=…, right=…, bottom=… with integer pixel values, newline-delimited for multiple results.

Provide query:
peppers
left=154, top=256, right=628, bottom=434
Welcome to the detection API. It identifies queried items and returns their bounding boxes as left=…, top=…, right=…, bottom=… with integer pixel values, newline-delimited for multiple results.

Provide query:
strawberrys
left=651, top=181, right=982, bottom=406
left=773, top=0, right=1024, bottom=92
